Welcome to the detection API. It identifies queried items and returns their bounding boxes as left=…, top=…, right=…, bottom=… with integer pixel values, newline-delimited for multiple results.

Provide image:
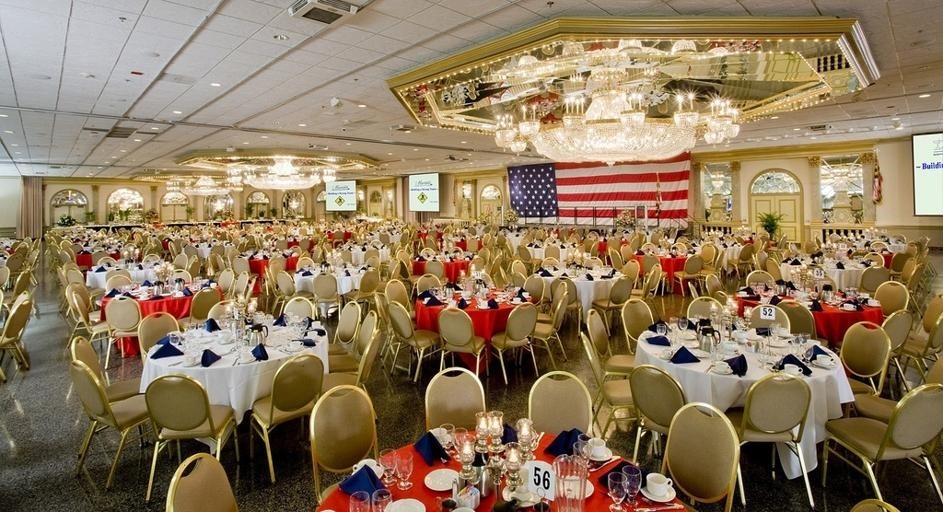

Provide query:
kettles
left=320, top=261, right=332, bottom=273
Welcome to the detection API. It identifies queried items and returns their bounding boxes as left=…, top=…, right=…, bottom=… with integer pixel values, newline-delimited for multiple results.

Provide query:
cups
left=114, top=276, right=214, bottom=300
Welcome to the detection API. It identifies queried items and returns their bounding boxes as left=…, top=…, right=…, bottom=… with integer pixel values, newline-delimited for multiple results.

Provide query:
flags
left=506, top=153, right=690, bottom=231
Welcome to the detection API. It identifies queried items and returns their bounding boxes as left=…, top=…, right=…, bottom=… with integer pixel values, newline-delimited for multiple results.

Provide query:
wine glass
left=165, top=309, right=309, bottom=368
left=755, top=274, right=883, bottom=312
left=348, top=409, right=680, bottom=512
left=658, top=310, right=837, bottom=380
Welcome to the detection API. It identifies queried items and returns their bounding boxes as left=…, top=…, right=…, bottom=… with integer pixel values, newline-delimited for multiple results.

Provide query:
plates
left=422, top=277, right=526, bottom=309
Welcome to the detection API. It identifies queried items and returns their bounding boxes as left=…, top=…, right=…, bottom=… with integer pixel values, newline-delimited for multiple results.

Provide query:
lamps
left=128, top=171, right=244, bottom=195
left=172, top=148, right=380, bottom=190
left=385, top=16, right=884, bottom=169
